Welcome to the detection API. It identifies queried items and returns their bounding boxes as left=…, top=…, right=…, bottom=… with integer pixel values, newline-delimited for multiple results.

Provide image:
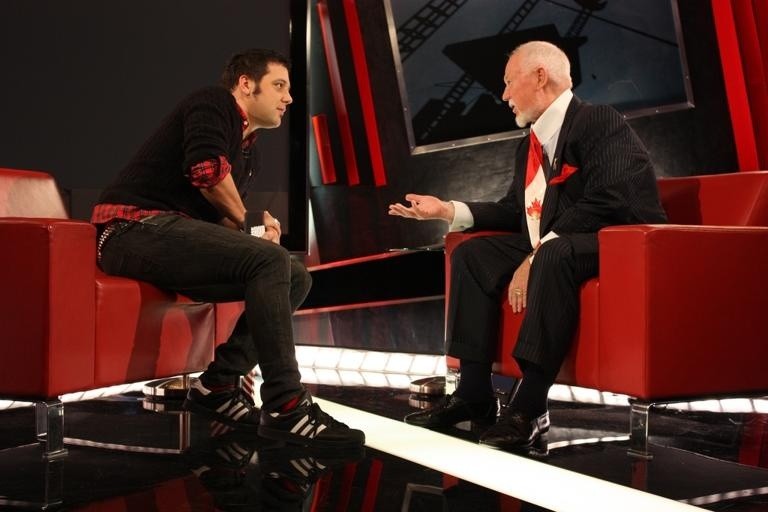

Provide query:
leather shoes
left=478, top=402, right=551, bottom=449
left=404, top=392, right=497, bottom=428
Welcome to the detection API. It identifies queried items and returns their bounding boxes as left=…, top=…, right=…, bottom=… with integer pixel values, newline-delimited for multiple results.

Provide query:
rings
left=514, top=289, right=522, bottom=296
left=274, top=219, right=278, bottom=224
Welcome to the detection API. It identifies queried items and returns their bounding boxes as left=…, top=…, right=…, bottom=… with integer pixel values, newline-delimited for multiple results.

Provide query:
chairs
left=0, top=168, right=254, bottom=463
left=444, top=170, right=768, bottom=460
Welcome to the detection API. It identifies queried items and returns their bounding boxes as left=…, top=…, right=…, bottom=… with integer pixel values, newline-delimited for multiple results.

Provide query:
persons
left=156, top=438, right=366, bottom=512
left=387, top=39, right=669, bottom=449
left=90, top=45, right=366, bottom=445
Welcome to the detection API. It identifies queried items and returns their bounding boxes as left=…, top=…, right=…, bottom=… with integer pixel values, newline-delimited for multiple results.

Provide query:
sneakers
left=248, top=446, right=367, bottom=500
left=181, top=376, right=262, bottom=430
left=173, top=429, right=259, bottom=478
left=257, top=389, right=366, bottom=450
